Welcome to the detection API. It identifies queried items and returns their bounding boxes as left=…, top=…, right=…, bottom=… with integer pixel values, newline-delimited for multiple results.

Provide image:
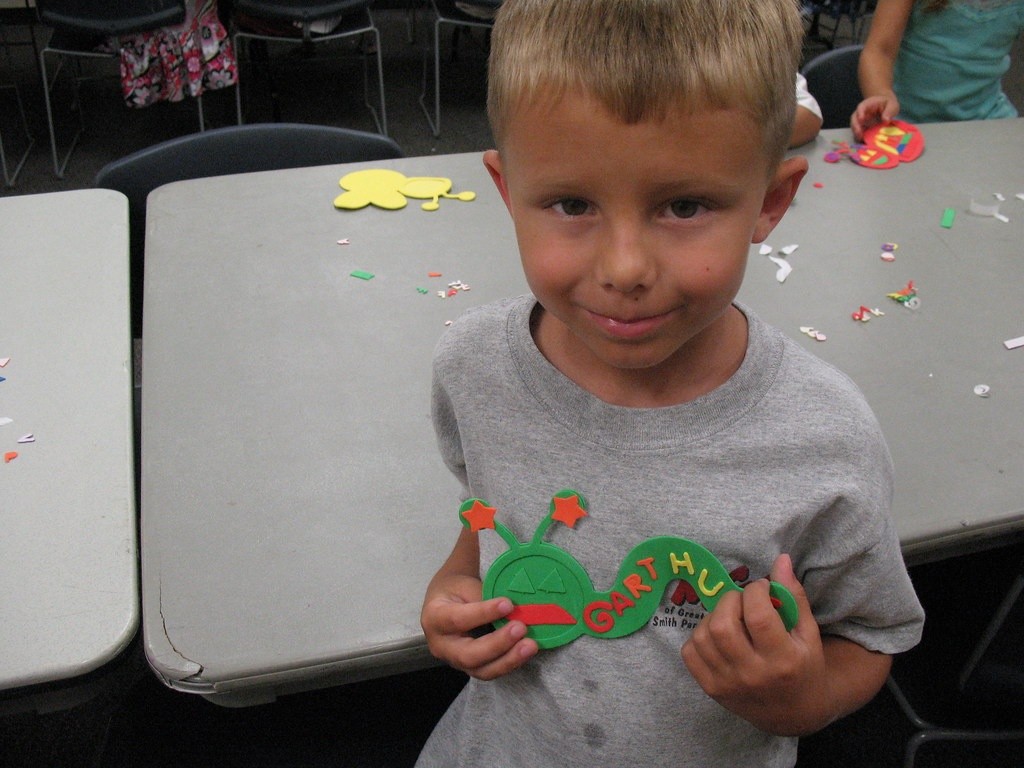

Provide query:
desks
left=140, top=115, right=1024, bottom=716
left=0, top=189, right=139, bottom=749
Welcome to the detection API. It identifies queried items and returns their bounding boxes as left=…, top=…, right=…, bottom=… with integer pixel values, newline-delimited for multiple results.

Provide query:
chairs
left=0, top=0, right=1024, bottom=768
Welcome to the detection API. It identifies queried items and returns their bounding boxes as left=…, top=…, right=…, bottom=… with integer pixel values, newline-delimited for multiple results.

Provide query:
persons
left=411, top=1, right=926, bottom=768
left=85, top=0, right=342, bottom=111
left=848, top=0, right=1024, bottom=142
left=785, top=63, right=823, bottom=150
